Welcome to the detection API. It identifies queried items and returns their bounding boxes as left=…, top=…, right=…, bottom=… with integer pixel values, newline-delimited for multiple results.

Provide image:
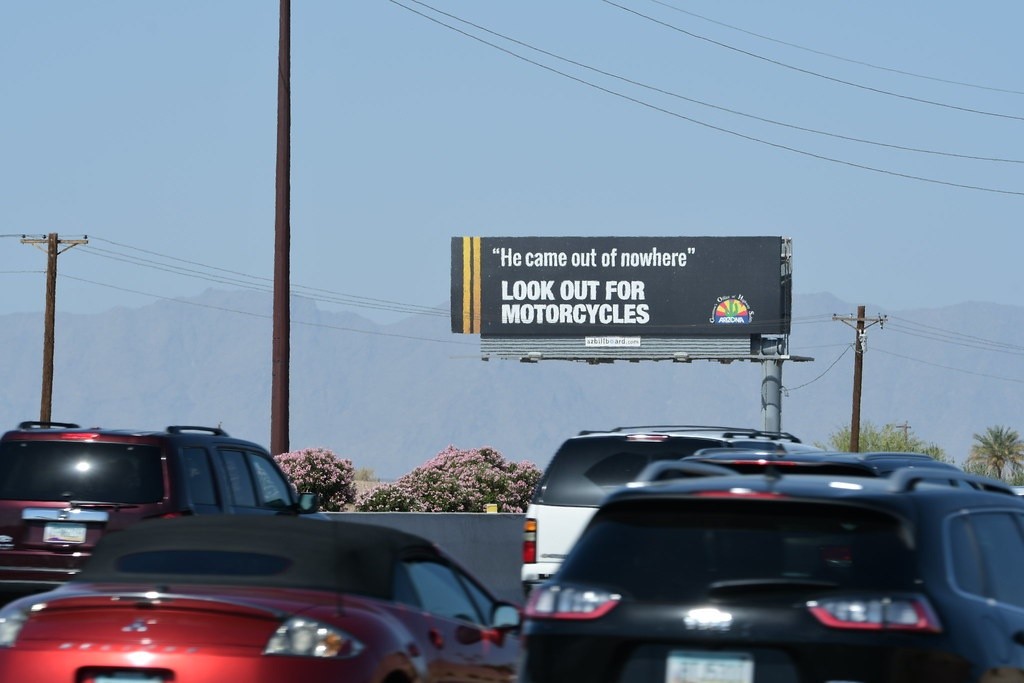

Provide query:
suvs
left=522, top=424, right=827, bottom=590
left=680, top=446, right=995, bottom=492
left=0, top=421, right=323, bottom=621
left=521, top=458, right=1023, bottom=683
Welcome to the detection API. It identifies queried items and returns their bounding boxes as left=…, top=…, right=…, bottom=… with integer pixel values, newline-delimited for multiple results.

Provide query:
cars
left=0, top=512, right=528, bottom=683
